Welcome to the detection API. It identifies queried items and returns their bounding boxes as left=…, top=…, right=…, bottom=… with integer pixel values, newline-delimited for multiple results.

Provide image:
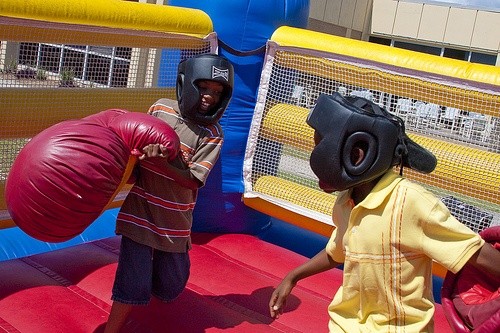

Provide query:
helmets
left=306, top=91, right=437, bottom=194
left=176, top=53, right=235, bottom=125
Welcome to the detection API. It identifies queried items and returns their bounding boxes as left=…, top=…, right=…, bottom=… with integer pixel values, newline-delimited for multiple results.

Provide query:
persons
left=89, top=54, right=234, bottom=333
left=266, top=89, right=500, bottom=333
